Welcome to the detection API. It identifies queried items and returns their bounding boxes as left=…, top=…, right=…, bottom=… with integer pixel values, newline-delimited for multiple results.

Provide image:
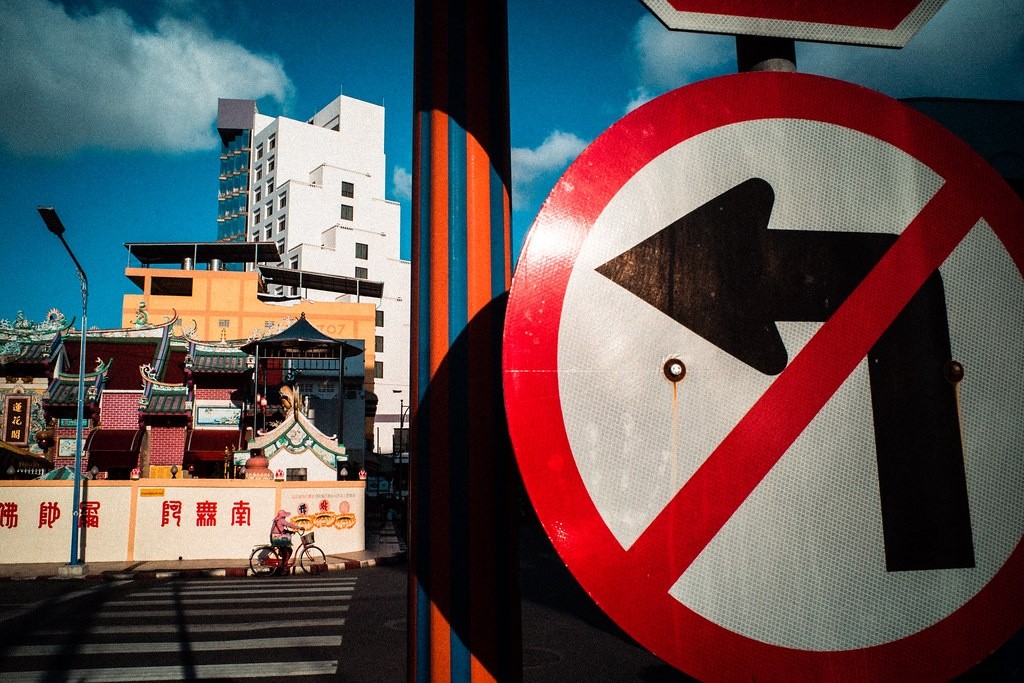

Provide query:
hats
left=272, top=510, right=291, bottom=521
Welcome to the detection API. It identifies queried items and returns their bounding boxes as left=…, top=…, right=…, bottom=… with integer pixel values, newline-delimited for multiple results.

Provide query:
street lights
left=36, top=202, right=95, bottom=565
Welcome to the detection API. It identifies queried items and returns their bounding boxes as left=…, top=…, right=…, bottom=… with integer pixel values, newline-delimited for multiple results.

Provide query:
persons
left=270, top=509, right=305, bottom=574
left=256, top=384, right=304, bottom=436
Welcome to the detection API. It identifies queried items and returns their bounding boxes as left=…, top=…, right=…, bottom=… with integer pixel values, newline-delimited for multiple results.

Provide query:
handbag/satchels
left=271, top=533, right=291, bottom=547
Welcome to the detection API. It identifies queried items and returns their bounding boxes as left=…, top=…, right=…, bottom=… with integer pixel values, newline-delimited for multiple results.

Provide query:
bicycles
left=251, top=527, right=329, bottom=578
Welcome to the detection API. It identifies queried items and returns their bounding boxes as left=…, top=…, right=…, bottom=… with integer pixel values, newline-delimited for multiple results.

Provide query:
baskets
left=302, top=532, right=315, bottom=545
